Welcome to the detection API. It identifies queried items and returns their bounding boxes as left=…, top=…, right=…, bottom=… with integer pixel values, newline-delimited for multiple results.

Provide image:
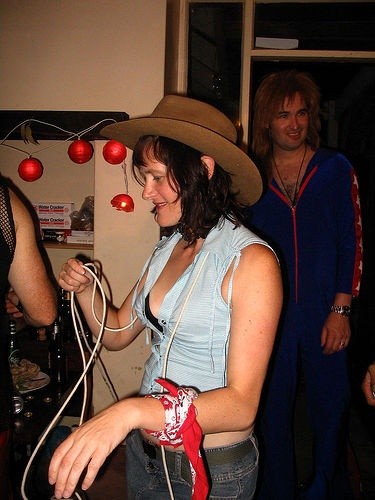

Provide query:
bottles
left=6, top=319, right=21, bottom=366
left=60, top=289, right=73, bottom=341
left=48, top=321, right=68, bottom=385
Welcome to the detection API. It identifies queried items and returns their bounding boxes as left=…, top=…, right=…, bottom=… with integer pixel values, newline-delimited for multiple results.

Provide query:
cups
left=13, top=396, right=23, bottom=415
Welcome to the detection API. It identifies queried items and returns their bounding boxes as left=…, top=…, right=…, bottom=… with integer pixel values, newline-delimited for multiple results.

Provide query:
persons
left=244, top=71, right=362, bottom=500
left=48, top=91, right=284, bottom=500
left=0, top=172, right=57, bottom=465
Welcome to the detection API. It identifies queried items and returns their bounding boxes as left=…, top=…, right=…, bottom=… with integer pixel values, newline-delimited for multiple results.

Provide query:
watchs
left=330, top=305, right=351, bottom=315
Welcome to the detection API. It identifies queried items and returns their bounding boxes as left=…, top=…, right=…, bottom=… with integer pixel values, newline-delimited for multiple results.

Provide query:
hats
left=99, top=95, right=263, bottom=207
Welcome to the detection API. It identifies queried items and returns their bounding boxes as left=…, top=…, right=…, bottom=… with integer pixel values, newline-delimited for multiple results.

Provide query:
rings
left=341, top=342, right=344, bottom=346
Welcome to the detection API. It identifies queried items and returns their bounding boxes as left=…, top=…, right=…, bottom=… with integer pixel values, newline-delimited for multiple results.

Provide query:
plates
left=12, top=366, right=50, bottom=394
